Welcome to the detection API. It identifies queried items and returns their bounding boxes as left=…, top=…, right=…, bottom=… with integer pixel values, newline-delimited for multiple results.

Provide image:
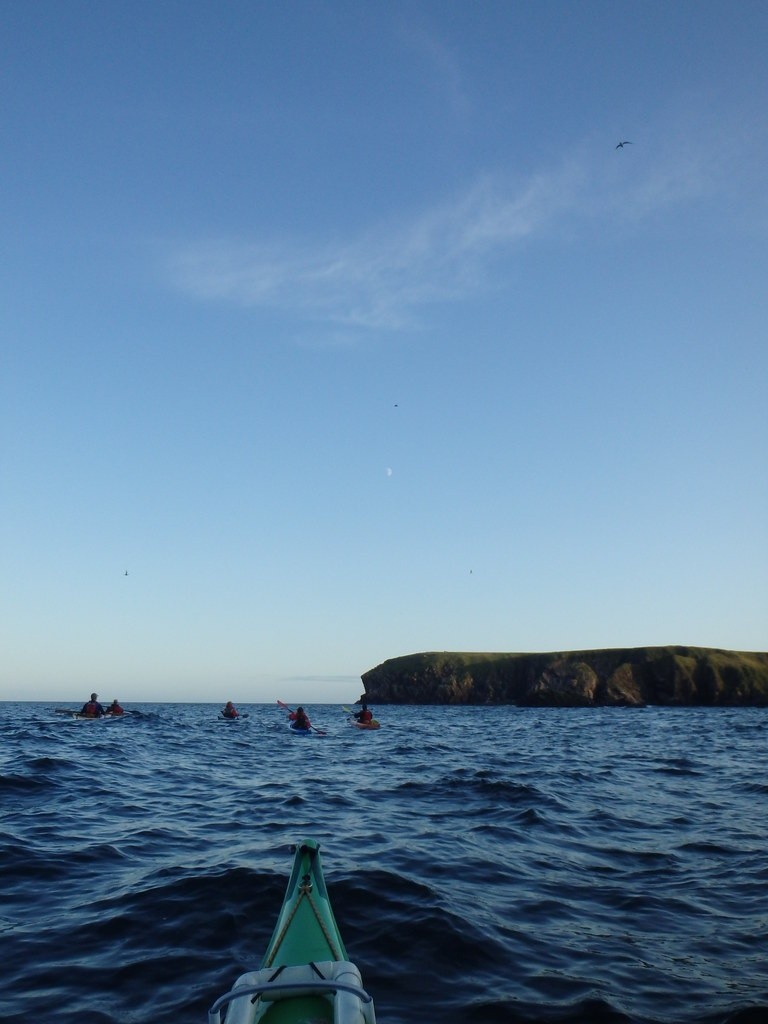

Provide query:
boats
left=351, top=719, right=381, bottom=730
left=72, top=713, right=104, bottom=721
left=106, top=711, right=130, bottom=719
left=217, top=715, right=239, bottom=720
left=287, top=720, right=313, bottom=735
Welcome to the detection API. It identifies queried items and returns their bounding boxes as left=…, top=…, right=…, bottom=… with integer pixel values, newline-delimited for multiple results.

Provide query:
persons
left=80, top=693, right=106, bottom=719
left=289, top=706, right=311, bottom=731
left=223, top=701, right=237, bottom=719
left=354, top=702, right=372, bottom=723
left=105, top=699, right=124, bottom=715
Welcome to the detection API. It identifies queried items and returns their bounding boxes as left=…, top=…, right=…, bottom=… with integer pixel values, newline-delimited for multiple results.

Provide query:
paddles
left=101, top=706, right=141, bottom=715
left=234, top=713, right=250, bottom=718
left=277, top=700, right=328, bottom=735
left=54, top=709, right=82, bottom=714
left=342, top=706, right=355, bottom=715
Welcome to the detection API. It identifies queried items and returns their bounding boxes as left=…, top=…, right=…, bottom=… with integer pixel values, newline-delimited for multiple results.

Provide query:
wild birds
left=614, top=141, right=635, bottom=150
